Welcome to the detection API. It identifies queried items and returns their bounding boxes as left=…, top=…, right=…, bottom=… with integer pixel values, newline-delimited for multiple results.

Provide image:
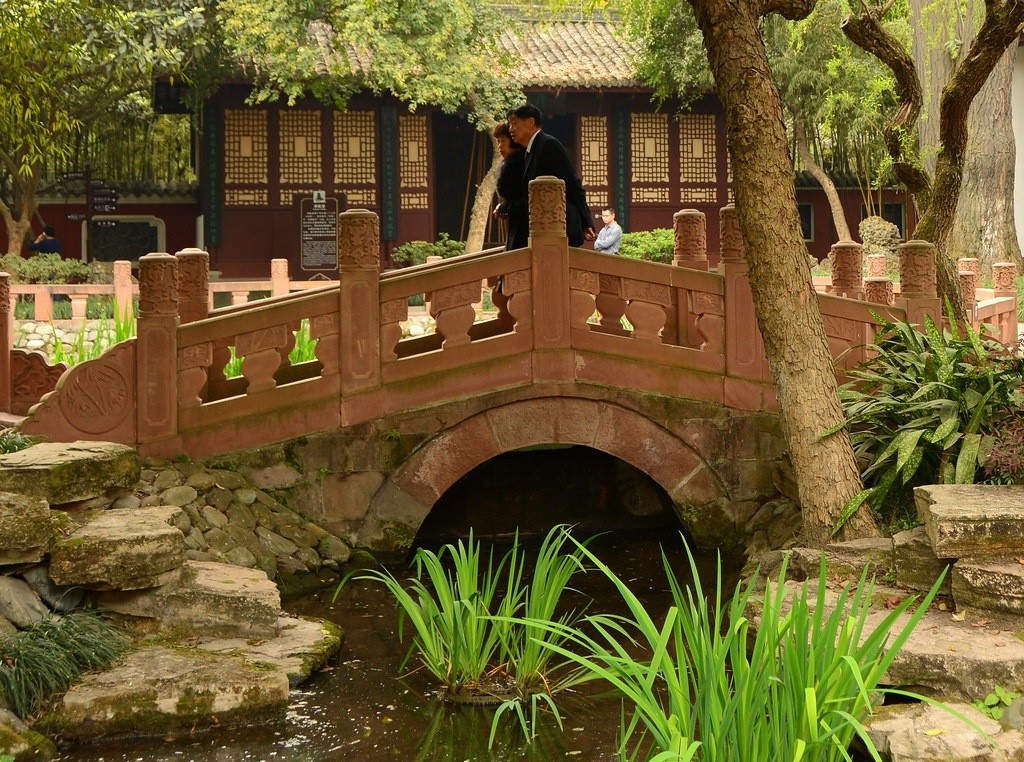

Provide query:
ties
left=522, top=152, right=529, bottom=170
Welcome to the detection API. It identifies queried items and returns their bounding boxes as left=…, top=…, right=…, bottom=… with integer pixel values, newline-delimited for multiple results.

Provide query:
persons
left=507, top=103, right=598, bottom=247
left=594, top=206, right=623, bottom=255
left=493, top=120, right=527, bottom=294
left=28, top=225, right=62, bottom=257
